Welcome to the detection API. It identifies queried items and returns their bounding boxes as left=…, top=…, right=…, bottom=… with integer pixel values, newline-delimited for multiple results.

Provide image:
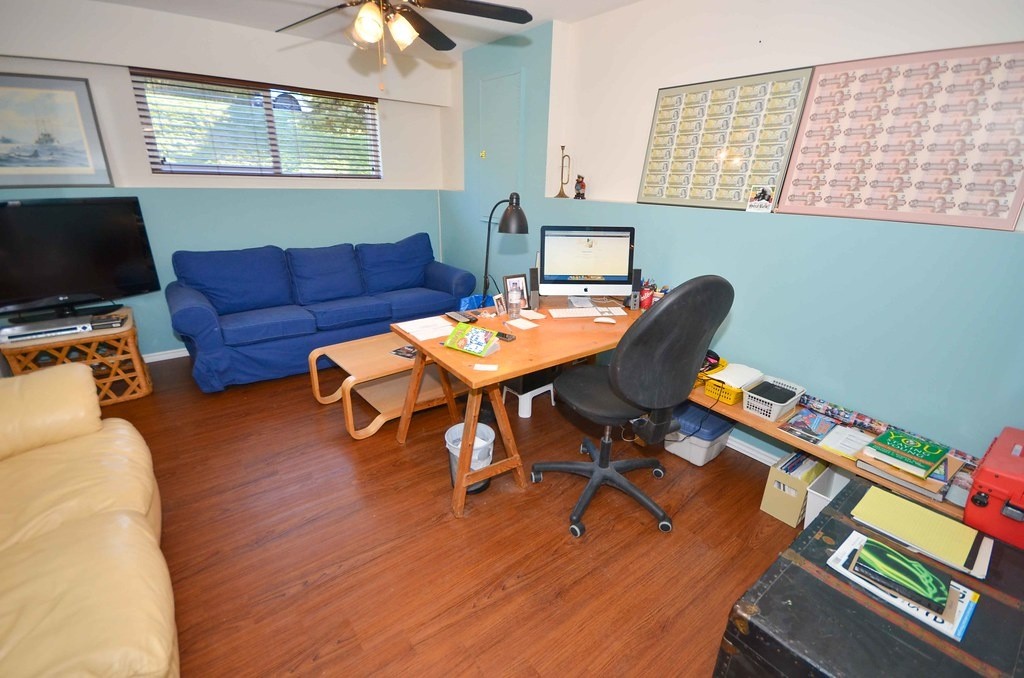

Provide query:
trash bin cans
left=444, top=422, right=496, bottom=494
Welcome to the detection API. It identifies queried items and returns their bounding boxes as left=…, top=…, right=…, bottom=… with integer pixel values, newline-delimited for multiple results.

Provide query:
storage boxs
left=741, top=374, right=807, bottom=422
left=963, top=426, right=1024, bottom=550
left=704, top=364, right=764, bottom=405
left=761, top=449, right=832, bottom=529
left=693, top=357, right=727, bottom=388
left=664, top=401, right=736, bottom=466
left=803, top=463, right=856, bottom=530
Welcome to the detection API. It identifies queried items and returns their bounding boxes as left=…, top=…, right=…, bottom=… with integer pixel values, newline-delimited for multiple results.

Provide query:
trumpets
left=554, top=145, right=572, bottom=198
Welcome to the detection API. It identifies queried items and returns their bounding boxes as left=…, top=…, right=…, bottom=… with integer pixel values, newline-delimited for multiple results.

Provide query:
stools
left=501, top=378, right=556, bottom=418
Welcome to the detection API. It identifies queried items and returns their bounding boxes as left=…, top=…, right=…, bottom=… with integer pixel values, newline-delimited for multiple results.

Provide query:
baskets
left=705, top=379, right=742, bottom=405
left=743, top=376, right=806, bottom=424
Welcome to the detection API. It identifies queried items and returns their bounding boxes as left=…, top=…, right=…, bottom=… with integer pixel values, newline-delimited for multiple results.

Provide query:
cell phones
left=496, top=332, right=516, bottom=341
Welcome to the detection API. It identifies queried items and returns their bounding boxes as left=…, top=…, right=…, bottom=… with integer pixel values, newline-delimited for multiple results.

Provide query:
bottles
left=507, top=282, right=522, bottom=319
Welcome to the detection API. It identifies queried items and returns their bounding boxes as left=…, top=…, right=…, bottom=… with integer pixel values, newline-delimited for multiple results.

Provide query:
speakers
left=630, top=269, right=642, bottom=310
left=529, top=268, right=539, bottom=309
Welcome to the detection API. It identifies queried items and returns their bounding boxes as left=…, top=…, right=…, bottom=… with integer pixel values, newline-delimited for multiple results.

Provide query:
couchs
left=0, top=363, right=181, bottom=678
left=164, top=233, right=476, bottom=394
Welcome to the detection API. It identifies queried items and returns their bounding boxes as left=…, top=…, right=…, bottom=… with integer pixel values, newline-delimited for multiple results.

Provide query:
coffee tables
left=307, top=331, right=469, bottom=439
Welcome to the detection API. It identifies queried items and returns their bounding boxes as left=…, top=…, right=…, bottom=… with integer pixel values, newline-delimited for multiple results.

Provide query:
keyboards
left=549, top=307, right=628, bottom=319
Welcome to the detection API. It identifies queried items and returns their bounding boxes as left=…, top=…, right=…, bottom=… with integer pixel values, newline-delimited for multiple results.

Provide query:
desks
left=390, top=296, right=649, bottom=518
left=712, top=474, right=1024, bottom=678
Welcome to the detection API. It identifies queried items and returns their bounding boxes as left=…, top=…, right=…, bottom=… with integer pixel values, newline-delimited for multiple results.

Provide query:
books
left=827, top=527, right=980, bottom=642
left=444, top=322, right=500, bottom=357
left=853, top=429, right=966, bottom=502
left=773, top=449, right=829, bottom=500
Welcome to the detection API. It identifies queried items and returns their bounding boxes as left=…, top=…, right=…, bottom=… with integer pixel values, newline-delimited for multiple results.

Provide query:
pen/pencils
left=641, top=278, right=656, bottom=286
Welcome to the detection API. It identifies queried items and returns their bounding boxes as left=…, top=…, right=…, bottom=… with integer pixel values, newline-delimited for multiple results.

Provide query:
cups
left=641, top=286, right=653, bottom=309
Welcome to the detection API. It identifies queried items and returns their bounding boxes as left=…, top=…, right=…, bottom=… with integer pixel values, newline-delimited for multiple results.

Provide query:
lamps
left=342, top=2, right=419, bottom=90
left=477, top=190, right=530, bottom=308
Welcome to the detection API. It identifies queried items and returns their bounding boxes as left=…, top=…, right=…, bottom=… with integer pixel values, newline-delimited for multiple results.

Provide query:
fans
left=275, top=0, right=534, bottom=52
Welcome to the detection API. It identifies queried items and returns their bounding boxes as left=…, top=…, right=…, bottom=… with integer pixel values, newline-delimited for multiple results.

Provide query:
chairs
left=530, top=275, right=734, bottom=540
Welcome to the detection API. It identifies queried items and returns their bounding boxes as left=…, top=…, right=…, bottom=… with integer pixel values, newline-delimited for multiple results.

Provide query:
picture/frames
left=0, top=72, right=114, bottom=189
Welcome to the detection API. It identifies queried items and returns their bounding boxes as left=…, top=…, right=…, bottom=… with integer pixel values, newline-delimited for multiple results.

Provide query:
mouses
left=593, top=317, right=616, bottom=324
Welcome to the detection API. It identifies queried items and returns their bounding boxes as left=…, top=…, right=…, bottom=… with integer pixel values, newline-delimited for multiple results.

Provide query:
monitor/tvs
left=0, top=196, right=161, bottom=324
left=539, top=225, right=636, bottom=308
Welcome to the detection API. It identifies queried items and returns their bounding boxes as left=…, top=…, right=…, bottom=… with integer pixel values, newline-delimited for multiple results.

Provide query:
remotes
left=445, top=311, right=478, bottom=323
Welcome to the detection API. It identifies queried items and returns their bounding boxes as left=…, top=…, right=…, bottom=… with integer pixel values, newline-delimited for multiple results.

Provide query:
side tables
left=0, top=306, right=153, bottom=407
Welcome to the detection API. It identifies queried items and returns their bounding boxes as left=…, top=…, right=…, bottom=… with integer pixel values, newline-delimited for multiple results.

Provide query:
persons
left=574, top=174, right=585, bottom=199
left=497, top=300, right=504, bottom=313
